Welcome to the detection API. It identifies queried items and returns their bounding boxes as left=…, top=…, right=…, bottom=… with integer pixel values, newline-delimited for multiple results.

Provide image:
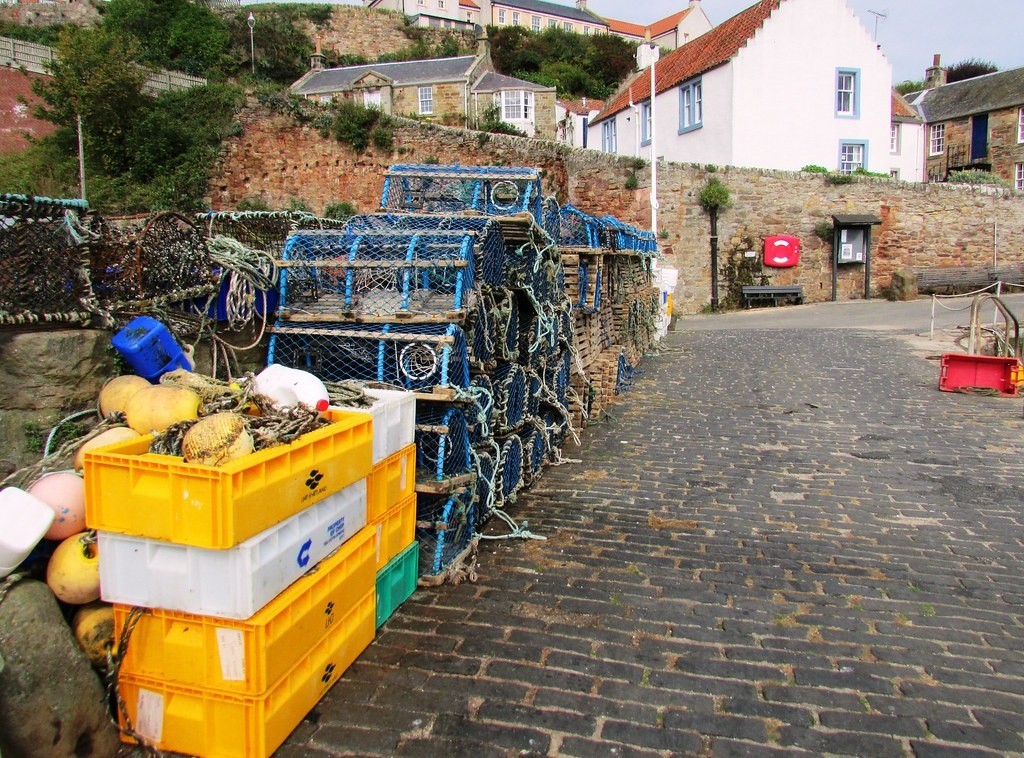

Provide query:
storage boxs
left=84, top=389, right=420, bottom=758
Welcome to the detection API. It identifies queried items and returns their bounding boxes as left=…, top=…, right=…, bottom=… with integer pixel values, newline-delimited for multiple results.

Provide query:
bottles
left=255, top=363, right=330, bottom=414
left=0, top=487, right=56, bottom=579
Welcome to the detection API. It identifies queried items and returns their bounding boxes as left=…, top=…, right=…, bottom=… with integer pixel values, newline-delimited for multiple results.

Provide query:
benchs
left=742, top=285, right=804, bottom=309
left=988, top=264, right=1024, bottom=293
left=913, top=267, right=990, bottom=295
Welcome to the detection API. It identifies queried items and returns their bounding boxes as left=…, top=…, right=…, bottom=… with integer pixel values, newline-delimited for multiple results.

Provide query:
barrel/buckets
left=111, top=316, right=193, bottom=385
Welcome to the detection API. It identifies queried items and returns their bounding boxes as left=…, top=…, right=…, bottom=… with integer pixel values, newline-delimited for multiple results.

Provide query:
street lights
left=246, top=10, right=258, bottom=79
